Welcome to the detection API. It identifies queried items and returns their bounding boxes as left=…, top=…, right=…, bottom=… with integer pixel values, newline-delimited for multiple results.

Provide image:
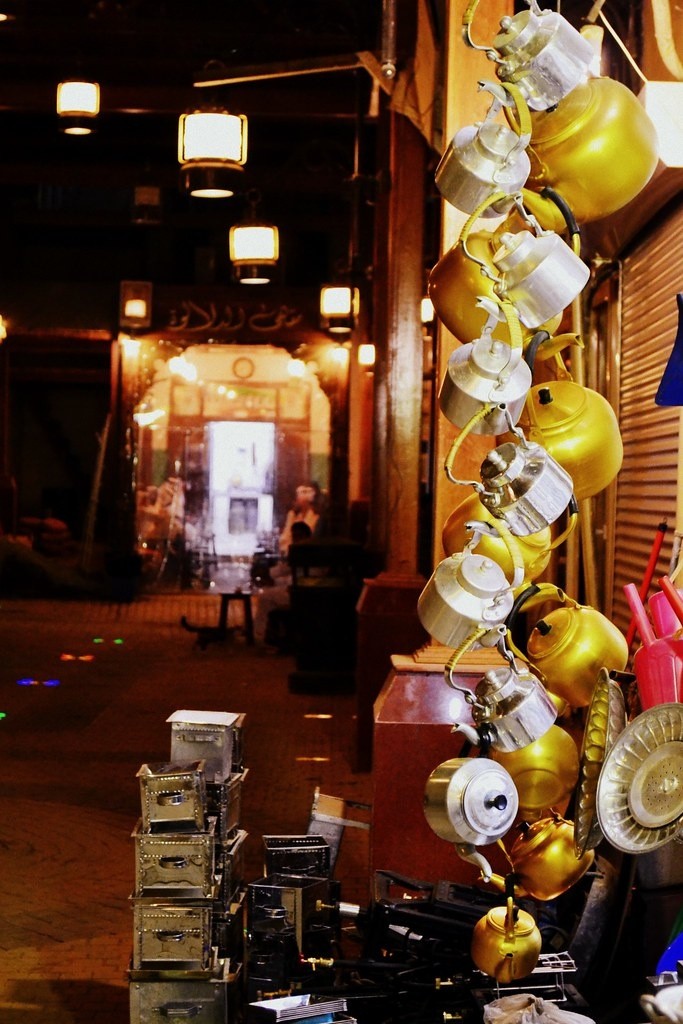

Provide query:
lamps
left=320, top=268, right=361, bottom=340
left=230, top=224, right=279, bottom=286
left=120, top=283, right=151, bottom=327
left=178, top=105, right=249, bottom=199
left=57, top=77, right=101, bottom=138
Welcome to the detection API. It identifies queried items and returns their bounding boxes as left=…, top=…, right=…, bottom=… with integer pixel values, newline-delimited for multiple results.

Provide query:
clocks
left=233, top=358, right=255, bottom=378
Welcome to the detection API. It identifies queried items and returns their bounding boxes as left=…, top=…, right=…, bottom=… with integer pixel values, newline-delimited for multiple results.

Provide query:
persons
left=311, top=483, right=329, bottom=536
left=277, top=480, right=322, bottom=554
left=251, top=520, right=324, bottom=649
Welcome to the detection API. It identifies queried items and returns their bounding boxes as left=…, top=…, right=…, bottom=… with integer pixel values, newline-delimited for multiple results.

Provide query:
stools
left=218, top=591, right=256, bottom=646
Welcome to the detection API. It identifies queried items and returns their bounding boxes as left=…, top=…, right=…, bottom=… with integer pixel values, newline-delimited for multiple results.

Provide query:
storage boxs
left=126, top=710, right=342, bottom=1024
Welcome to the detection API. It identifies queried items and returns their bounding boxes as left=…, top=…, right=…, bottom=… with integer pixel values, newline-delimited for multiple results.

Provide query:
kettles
left=416, top=1, right=659, bottom=983
